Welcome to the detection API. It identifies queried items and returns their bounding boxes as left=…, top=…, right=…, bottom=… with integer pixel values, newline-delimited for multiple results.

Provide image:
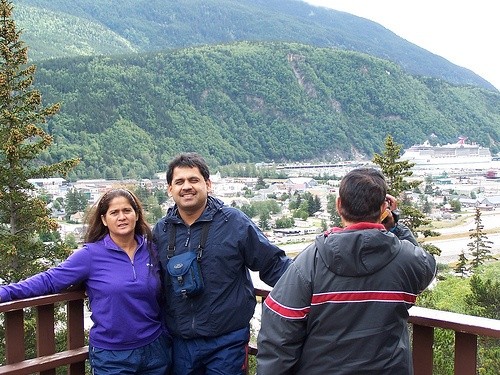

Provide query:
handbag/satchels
left=167, top=223, right=211, bottom=299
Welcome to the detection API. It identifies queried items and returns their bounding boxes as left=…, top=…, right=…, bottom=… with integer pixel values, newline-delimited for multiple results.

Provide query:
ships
left=396, top=140, right=493, bottom=165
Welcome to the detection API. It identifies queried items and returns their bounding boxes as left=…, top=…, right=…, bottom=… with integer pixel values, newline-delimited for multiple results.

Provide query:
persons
left=255, top=166, right=438, bottom=375
left=0, top=188, right=172, bottom=375
left=67, top=152, right=295, bottom=375
